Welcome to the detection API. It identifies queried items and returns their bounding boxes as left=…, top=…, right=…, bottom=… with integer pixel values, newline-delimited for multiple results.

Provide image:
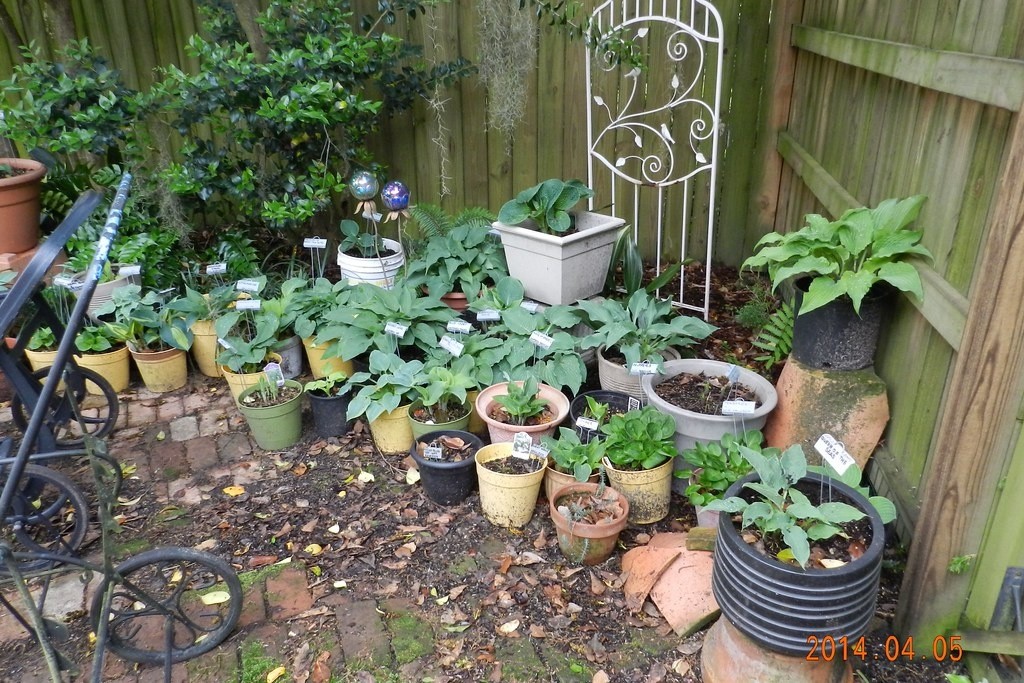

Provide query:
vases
left=552, top=483, right=630, bottom=566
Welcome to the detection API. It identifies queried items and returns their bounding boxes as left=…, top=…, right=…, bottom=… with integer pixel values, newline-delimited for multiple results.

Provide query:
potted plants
left=1, top=158, right=934, bottom=659
